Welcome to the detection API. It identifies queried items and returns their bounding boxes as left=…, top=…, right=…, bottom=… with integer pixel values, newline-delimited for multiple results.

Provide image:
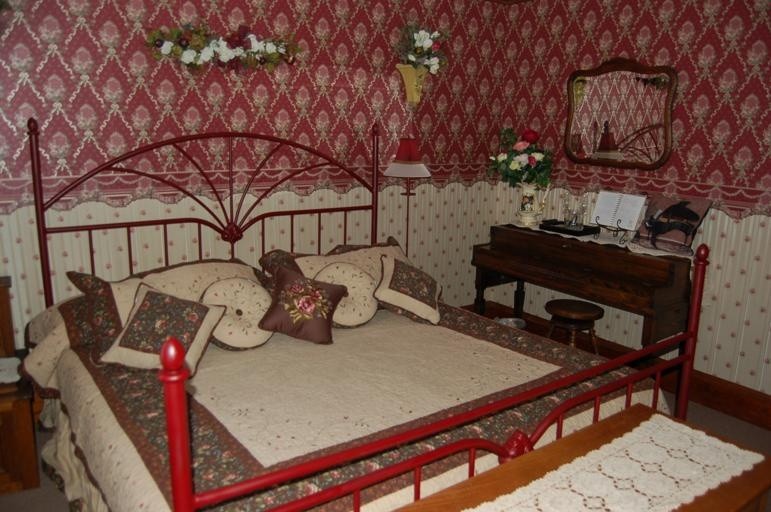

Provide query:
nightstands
left=1, top=274, right=42, bottom=495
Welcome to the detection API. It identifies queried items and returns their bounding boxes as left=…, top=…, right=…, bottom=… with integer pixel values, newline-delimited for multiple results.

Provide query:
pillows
left=375, top=250, right=447, bottom=328
left=198, top=276, right=273, bottom=352
left=312, top=261, right=376, bottom=330
left=61, top=255, right=273, bottom=352
left=100, top=282, right=228, bottom=378
left=260, top=265, right=347, bottom=344
left=259, top=235, right=412, bottom=281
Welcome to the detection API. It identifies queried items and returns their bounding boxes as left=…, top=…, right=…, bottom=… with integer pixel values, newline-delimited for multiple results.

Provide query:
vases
left=393, top=64, right=430, bottom=106
left=511, top=182, right=552, bottom=227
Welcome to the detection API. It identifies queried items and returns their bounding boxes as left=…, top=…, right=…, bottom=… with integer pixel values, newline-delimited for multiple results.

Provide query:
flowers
left=484, top=126, right=559, bottom=189
left=145, top=18, right=297, bottom=76
left=402, top=25, right=448, bottom=75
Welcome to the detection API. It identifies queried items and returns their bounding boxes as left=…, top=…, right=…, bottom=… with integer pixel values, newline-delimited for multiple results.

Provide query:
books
left=589, top=189, right=647, bottom=232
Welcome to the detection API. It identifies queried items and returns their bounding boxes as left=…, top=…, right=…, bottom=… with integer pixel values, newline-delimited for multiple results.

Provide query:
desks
left=389, top=401, right=769, bottom=511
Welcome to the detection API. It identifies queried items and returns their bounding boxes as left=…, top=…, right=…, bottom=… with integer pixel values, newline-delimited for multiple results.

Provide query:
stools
left=544, top=298, right=605, bottom=355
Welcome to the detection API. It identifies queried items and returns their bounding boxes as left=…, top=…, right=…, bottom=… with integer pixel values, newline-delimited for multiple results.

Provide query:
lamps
left=592, top=132, right=623, bottom=163
left=382, top=134, right=431, bottom=256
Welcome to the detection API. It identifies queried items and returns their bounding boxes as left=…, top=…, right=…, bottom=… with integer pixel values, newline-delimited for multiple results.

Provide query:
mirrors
left=566, top=58, right=677, bottom=169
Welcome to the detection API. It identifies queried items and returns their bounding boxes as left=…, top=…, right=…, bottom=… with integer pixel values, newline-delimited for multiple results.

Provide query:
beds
left=605, top=118, right=664, bottom=164
left=22, top=110, right=711, bottom=512
